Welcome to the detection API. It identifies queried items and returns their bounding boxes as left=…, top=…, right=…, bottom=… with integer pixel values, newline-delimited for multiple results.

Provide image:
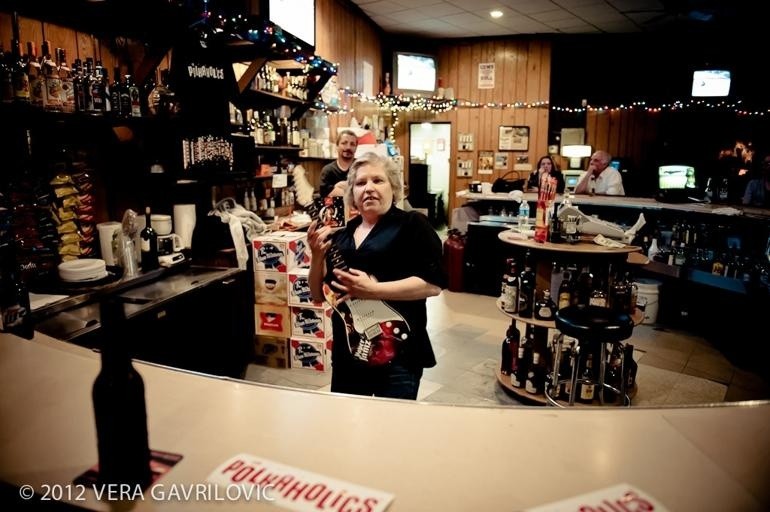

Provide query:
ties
left=658, top=164, right=696, bottom=192
left=690, top=69, right=733, bottom=100
left=260, top=0, right=318, bottom=48
left=392, top=50, right=437, bottom=100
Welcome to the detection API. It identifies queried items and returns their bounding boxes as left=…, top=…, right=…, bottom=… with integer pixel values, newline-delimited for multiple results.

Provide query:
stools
left=545, top=306, right=634, bottom=408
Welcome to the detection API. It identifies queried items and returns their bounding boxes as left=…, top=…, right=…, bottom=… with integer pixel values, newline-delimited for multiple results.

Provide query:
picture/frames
left=498, top=126, right=530, bottom=152
left=477, top=150, right=494, bottom=175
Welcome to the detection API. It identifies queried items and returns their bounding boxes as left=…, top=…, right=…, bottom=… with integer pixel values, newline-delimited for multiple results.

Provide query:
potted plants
left=498, top=126, right=530, bottom=152
left=477, top=150, right=494, bottom=175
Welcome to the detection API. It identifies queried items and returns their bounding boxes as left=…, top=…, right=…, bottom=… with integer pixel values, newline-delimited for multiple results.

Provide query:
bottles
left=89, top=285, right=154, bottom=494
left=496, top=199, right=767, bottom=404
left=2, top=40, right=179, bottom=117
left=228, top=59, right=309, bottom=207
left=436, top=77, right=445, bottom=99
left=139, top=206, right=160, bottom=272
left=0, top=239, right=34, bottom=340
left=382, top=73, right=391, bottom=95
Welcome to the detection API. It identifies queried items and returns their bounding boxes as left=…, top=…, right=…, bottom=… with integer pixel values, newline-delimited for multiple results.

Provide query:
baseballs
left=443, top=228, right=459, bottom=286
left=447, top=231, right=467, bottom=292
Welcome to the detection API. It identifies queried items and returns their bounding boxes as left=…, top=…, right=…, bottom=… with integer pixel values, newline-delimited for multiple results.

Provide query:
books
left=251, top=230, right=333, bottom=372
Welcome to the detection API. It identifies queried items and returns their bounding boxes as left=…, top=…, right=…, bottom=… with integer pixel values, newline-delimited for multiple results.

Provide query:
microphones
left=545, top=306, right=634, bottom=408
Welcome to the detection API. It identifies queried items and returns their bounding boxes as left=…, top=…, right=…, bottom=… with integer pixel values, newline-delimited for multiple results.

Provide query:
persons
left=574, top=150, right=625, bottom=196
left=320, top=131, right=357, bottom=199
left=742, top=153, right=770, bottom=209
left=308, top=152, right=449, bottom=400
left=527, top=156, right=565, bottom=193
left=478, top=159, right=493, bottom=170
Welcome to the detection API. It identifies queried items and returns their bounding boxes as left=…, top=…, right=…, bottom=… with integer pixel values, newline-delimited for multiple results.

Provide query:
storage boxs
left=251, top=230, right=333, bottom=372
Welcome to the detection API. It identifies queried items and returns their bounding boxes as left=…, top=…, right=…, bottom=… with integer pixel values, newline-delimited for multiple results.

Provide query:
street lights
left=492, top=178, right=526, bottom=192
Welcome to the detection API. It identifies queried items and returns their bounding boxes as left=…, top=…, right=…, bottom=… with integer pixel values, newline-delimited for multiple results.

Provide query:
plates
left=495, top=230, right=645, bottom=403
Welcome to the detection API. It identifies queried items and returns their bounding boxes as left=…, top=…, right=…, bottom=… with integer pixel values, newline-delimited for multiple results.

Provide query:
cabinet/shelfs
left=139, top=206, right=160, bottom=272
left=382, top=73, right=391, bottom=95
left=0, top=42, right=337, bottom=268
left=436, top=77, right=445, bottom=99
left=89, top=285, right=154, bottom=494
left=495, top=230, right=645, bottom=403
left=0, top=239, right=34, bottom=340
